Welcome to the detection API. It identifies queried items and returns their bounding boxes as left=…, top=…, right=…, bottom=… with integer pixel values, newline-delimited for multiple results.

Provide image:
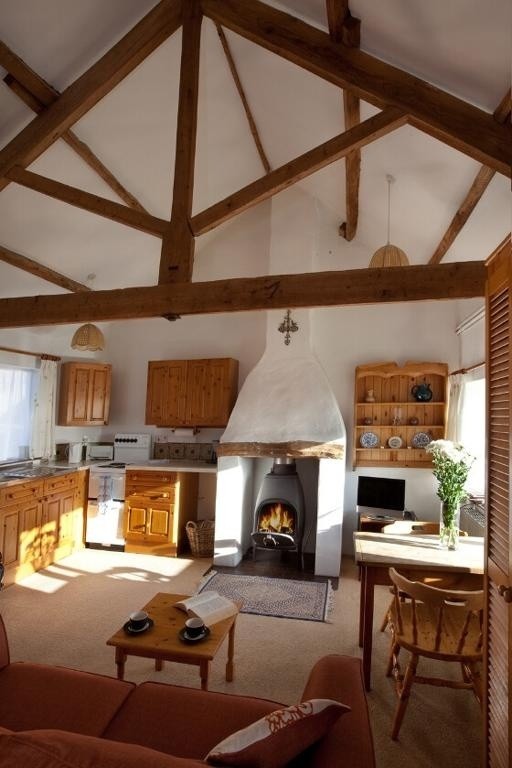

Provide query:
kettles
left=64, top=442, right=82, bottom=464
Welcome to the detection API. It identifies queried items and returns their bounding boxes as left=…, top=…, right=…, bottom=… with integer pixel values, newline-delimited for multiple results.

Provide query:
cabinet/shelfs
left=55, top=362, right=112, bottom=428
left=352, top=359, right=449, bottom=471
left=122, top=469, right=200, bottom=559
left=0, top=479, right=44, bottom=594
left=45, top=469, right=83, bottom=572
left=145, top=360, right=186, bottom=426
left=187, top=356, right=240, bottom=425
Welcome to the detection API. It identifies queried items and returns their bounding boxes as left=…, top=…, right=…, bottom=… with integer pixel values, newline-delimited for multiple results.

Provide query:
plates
left=389, top=437, right=402, bottom=448
left=410, top=432, right=431, bottom=448
left=178, top=626, right=210, bottom=643
left=359, top=432, right=378, bottom=448
left=124, top=619, right=152, bottom=633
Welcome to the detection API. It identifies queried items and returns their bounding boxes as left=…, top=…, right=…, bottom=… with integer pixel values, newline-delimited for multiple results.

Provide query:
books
left=171, top=587, right=239, bottom=630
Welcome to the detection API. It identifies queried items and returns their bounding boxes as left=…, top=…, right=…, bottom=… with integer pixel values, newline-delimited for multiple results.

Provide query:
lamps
left=368, top=173, right=412, bottom=268
left=71, top=274, right=108, bottom=351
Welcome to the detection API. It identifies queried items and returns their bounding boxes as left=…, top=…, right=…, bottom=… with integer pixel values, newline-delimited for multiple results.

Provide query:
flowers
left=425, top=437, right=478, bottom=541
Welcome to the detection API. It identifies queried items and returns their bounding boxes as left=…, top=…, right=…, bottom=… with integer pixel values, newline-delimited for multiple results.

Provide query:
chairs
left=382, top=517, right=487, bottom=743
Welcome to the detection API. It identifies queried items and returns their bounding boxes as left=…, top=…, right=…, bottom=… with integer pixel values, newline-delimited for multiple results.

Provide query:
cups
left=129, top=610, right=148, bottom=629
left=185, top=618, right=207, bottom=637
left=33, top=459, right=41, bottom=468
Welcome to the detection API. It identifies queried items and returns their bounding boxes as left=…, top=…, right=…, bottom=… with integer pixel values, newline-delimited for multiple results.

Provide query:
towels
left=98, top=475, right=115, bottom=516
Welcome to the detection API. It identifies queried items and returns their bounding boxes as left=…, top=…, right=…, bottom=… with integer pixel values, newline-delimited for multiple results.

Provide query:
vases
left=439, top=501, right=459, bottom=550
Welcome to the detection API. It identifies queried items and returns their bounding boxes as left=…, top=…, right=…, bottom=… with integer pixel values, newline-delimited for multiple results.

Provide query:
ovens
left=86, top=470, right=125, bottom=546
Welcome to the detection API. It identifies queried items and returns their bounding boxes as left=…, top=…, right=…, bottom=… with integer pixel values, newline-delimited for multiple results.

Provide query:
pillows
left=202, top=696, right=354, bottom=767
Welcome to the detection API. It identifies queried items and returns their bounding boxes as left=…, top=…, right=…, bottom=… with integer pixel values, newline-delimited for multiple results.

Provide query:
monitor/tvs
left=355, top=475, right=406, bottom=522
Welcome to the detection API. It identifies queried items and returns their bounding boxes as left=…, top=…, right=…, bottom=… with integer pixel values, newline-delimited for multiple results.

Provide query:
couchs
left=0, top=611, right=377, bottom=767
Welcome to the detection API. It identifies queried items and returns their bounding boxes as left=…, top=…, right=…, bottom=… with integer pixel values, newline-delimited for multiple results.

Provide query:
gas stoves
left=90, top=461, right=143, bottom=469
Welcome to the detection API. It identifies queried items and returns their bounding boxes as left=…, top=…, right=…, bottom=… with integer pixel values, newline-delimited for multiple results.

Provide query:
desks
left=351, top=529, right=487, bottom=693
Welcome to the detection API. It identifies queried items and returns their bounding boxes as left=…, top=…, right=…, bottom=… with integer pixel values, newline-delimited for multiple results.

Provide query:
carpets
left=193, top=569, right=337, bottom=626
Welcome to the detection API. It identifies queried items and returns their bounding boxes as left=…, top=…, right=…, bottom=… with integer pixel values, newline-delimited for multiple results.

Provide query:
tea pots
left=411, top=383, right=434, bottom=401
